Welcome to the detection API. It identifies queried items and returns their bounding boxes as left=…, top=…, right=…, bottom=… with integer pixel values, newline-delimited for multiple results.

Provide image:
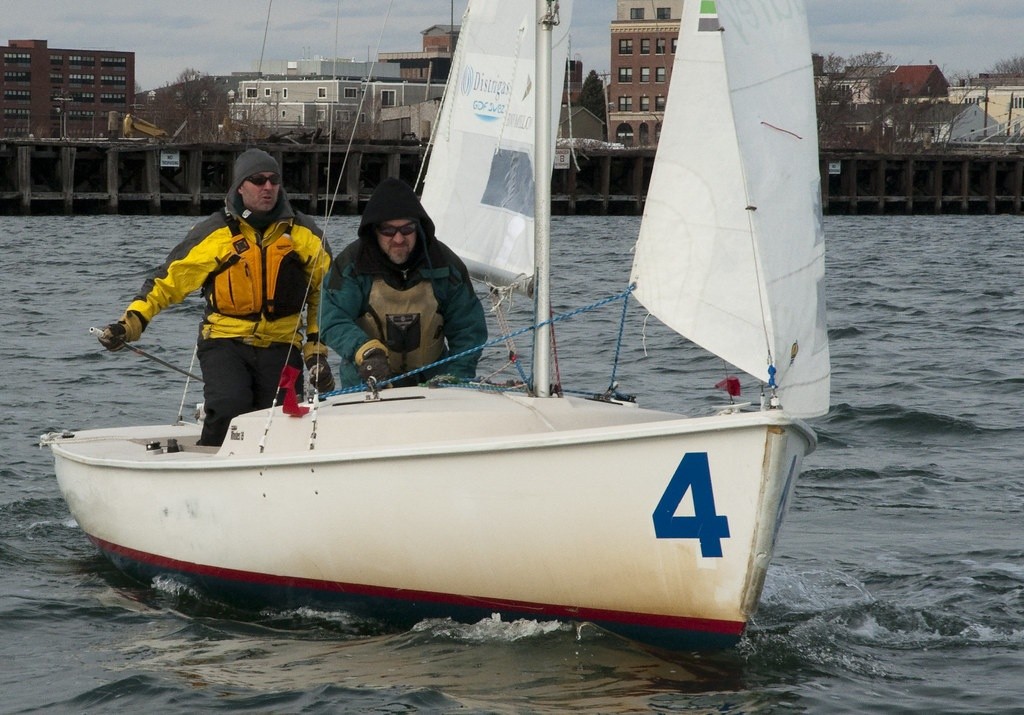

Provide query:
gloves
left=98, top=310, right=143, bottom=352
left=306, top=355, right=335, bottom=394
left=358, top=348, right=392, bottom=384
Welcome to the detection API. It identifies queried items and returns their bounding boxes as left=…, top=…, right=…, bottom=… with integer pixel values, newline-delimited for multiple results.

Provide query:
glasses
left=245, top=173, right=281, bottom=185
left=374, top=222, right=417, bottom=237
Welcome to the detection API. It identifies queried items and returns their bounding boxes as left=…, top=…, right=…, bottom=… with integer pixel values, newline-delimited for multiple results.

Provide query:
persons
left=90, top=147, right=336, bottom=446
left=317, top=178, right=489, bottom=390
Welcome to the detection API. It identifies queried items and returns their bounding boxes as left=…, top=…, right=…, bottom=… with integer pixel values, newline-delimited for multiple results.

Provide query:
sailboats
left=33, top=0, right=833, bottom=657
